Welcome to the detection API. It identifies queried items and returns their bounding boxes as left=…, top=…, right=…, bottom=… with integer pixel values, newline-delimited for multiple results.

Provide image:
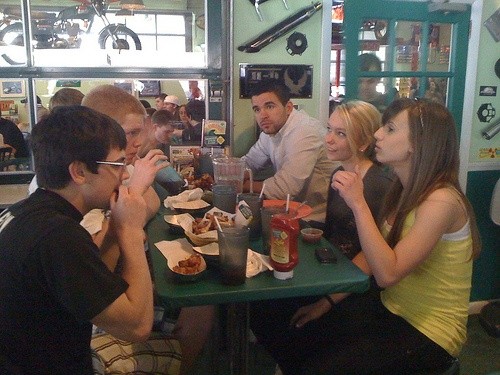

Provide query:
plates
left=263, top=200, right=312, bottom=220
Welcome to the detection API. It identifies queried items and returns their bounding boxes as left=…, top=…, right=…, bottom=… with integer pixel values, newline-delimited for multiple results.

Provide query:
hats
left=164, top=95, right=182, bottom=107
left=21, top=95, right=42, bottom=108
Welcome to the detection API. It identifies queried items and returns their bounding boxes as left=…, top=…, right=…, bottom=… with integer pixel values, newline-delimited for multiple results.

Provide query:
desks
left=146, top=197, right=372, bottom=375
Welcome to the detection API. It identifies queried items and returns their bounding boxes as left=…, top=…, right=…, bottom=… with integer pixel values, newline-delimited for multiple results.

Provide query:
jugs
left=212, top=158, right=254, bottom=205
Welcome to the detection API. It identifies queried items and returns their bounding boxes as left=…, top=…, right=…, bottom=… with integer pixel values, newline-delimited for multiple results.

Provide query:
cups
left=154, top=159, right=186, bottom=196
left=213, top=185, right=235, bottom=213
left=217, top=225, right=250, bottom=286
left=235, top=193, right=263, bottom=242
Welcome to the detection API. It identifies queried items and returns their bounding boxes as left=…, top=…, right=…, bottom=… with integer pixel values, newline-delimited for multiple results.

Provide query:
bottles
left=269, top=214, right=299, bottom=280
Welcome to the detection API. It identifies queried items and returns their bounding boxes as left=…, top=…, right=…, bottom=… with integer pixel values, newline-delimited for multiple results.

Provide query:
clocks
left=197, top=15, right=205, bottom=30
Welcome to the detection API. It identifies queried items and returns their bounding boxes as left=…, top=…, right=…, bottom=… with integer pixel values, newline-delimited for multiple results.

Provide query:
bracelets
left=325, top=294, right=337, bottom=307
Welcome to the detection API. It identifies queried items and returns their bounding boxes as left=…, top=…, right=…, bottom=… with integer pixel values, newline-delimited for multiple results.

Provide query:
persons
left=249, top=100, right=393, bottom=366
left=221, top=78, right=343, bottom=236
left=275, top=97, right=482, bottom=375
left=131, top=93, right=230, bottom=167
left=329, top=53, right=446, bottom=117
left=0, top=105, right=154, bottom=375
left=188, top=81, right=203, bottom=102
left=49, top=88, right=85, bottom=114
left=489, top=177, right=500, bottom=226
left=28, top=84, right=182, bottom=375
left=0, top=96, right=48, bottom=171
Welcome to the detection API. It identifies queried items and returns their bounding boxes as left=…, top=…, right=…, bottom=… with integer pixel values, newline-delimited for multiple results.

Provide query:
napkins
left=245, top=248, right=275, bottom=278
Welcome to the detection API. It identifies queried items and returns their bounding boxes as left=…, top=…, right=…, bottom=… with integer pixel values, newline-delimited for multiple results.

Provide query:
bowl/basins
left=300, top=228, right=323, bottom=244
left=167, top=254, right=207, bottom=283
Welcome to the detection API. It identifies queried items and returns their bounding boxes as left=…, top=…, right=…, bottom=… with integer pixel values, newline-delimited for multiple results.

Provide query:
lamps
left=115, top=0, right=145, bottom=16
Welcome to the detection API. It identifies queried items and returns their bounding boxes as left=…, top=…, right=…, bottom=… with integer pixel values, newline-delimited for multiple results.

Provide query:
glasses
left=94, top=160, right=127, bottom=173
